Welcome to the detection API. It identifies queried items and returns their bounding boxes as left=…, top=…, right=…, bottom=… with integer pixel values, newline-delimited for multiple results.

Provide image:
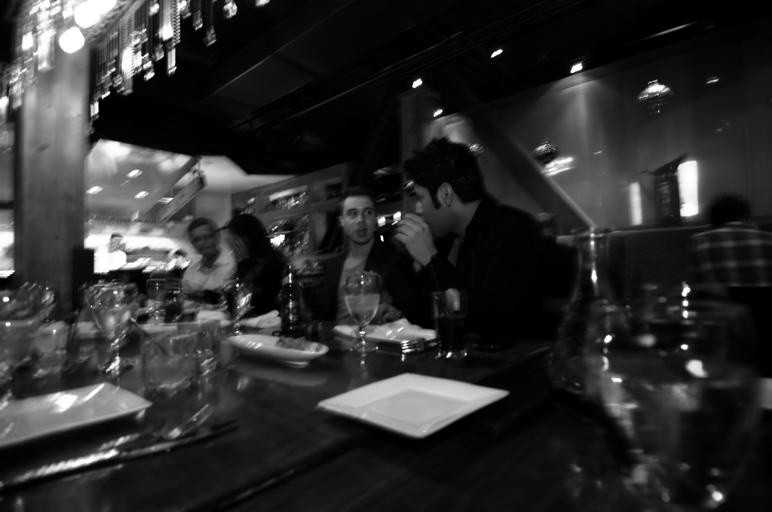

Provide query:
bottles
left=548, top=220, right=634, bottom=393
left=281, top=265, right=301, bottom=327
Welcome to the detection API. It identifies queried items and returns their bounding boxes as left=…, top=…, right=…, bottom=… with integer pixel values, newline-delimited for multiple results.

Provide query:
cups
left=0, top=283, right=54, bottom=384
left=429, top=288, right=470, bottom=358
left=165, top=288, right=184, bottom=321
left=137, top=321, right=224, bottom=397
left=576, top=292, right=763, bottom=512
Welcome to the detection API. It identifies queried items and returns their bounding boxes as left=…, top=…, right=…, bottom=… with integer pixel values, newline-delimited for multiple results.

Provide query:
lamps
left=637, top=78, right=673, bottom=116
left=532, top=140, right=559, bottom=166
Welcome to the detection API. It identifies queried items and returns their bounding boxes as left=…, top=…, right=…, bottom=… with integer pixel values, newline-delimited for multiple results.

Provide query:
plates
left=227, top=333, right=333, bottom=369
left=1, top=380, right=155, bottom=450
left=334, top=317, right=439, bottom=345
left=314, top=372, right=513, bottom=441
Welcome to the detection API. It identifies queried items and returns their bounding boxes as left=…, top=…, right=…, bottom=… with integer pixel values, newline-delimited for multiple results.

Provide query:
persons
left=317, top=188, right=398, bottom=321
left=687, top=190, right=772, bottom=456
left=221, top=212, right=289, bottom=309
left=93, top=233, right=129, bottom=273
left=178, top=215, right=240, bottom=294
left=391, top=132, right=547, bottom=340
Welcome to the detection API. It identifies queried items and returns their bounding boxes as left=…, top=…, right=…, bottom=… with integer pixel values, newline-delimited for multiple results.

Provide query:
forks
left=1, top=404, right=220, bottom=492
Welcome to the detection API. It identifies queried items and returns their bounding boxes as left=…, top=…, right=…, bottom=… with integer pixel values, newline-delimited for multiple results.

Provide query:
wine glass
left=341, top=273, right=383, bottom=356
left=147, top=277, right=167, bottom=316
left=221, top=278, right=253, bottom=336
left=88, top=280, right=136, bottom=374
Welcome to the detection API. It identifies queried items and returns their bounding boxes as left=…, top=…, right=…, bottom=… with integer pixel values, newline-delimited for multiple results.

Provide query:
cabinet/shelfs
left=231, top=160, right=373, bottom=280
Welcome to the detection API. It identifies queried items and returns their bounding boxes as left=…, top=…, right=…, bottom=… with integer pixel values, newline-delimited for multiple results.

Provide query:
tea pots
left=636, top=151, right=688, bottom=227
